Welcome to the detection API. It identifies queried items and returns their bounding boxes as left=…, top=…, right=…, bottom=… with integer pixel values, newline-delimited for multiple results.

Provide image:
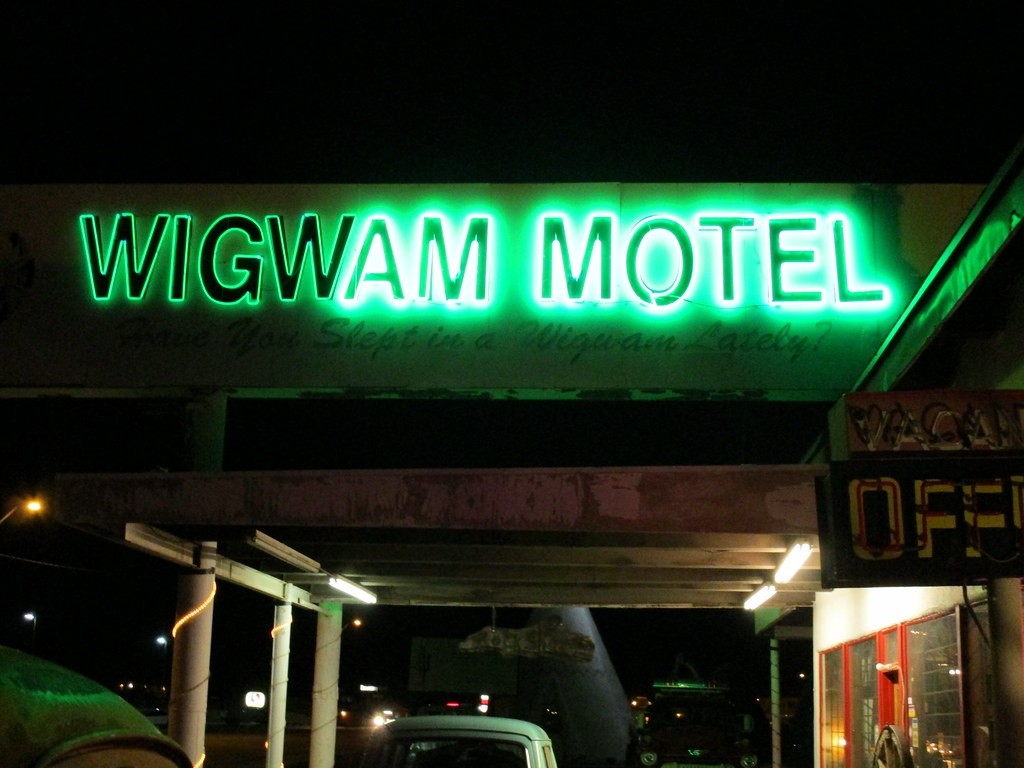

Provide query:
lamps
left=742, top=583, right=777, bottom=610
left=247, top=530, right=320, bottom=574
left=772, top=538, right=812, bottom=584
left=328, top=573, right=377, bottom=604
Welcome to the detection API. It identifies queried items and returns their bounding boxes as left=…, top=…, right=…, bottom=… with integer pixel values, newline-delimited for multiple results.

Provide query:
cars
left=361, top=715, right=557, bottom=768
left=631, top=696, right=651, bottom=710
left=339, top=700, right=409, bottom=727
left=629, top=687, right=772, bottom=768
